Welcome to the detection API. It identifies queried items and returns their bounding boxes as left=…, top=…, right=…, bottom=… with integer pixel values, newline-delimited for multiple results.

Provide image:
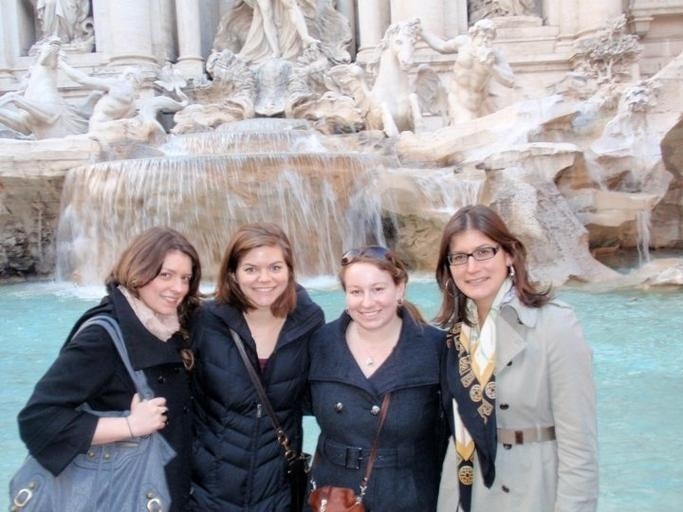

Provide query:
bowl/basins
left=348, top=324, right=394, bottom=366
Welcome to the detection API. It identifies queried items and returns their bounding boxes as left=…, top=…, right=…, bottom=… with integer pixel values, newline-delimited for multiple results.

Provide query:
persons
left=15, top=223, right=200, bottom=512
left=434, top=202, right=602, bottom=512
left=177, top=219, right=325, bottom=511
left=294, top=244, right=455, bottom=511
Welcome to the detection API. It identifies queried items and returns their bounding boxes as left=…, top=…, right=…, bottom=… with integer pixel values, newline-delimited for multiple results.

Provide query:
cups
left=441, top=239, right=502, bottom=266
left=341, top=247, right=388, bottom=266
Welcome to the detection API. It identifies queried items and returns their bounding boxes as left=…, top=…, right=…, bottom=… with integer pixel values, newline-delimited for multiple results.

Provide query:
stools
left=495, top=426, right=556, bottom=445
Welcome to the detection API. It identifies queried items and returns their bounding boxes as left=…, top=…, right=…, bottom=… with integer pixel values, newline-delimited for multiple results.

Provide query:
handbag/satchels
left=123, top=415, right=134, bottom=442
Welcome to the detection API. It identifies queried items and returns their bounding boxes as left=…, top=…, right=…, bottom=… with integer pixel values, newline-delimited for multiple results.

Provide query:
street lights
left=305, top=486, right=364, bottom=512
left=284, top=451, right=309, bottom=512
left=4, top=409, right=177, bottom=512
